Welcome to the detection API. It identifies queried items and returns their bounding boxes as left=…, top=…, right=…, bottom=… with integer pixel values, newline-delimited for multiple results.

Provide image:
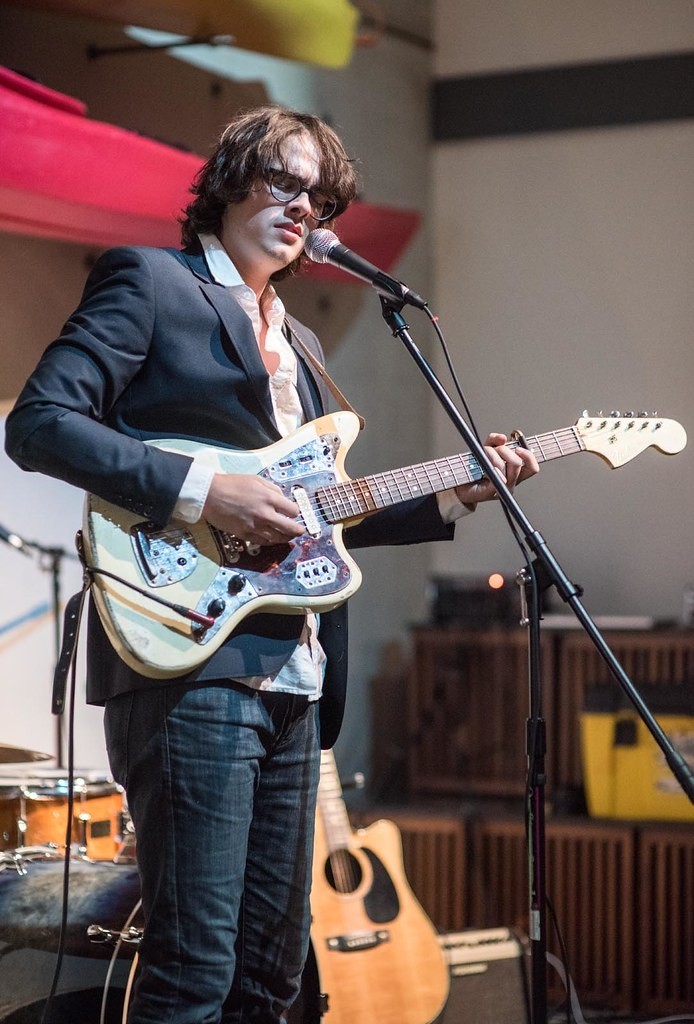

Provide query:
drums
left=22, top=778, right=125, bottom=863
left=0, top=785, right=23, bottom=853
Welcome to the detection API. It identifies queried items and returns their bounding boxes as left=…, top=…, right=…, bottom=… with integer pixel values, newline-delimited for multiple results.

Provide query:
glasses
left=268, top=167, right=337, bottom=221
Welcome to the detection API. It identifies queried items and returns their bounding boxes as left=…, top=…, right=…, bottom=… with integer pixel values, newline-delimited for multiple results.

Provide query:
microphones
left=304, top=228, right=429, bottom=310
left=0, top=524, right=25, bottom=551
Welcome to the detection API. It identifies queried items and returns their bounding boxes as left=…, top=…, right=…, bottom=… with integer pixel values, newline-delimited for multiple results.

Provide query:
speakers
left=431, top=923, right=533, bottom=1024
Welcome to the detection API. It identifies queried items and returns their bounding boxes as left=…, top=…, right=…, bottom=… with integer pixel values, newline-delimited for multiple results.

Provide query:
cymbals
left=0, top=739, right=56, bottom=768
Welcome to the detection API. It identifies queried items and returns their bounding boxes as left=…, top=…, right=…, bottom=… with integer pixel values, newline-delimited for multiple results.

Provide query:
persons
left=3, top=104, right=539, bottom=1024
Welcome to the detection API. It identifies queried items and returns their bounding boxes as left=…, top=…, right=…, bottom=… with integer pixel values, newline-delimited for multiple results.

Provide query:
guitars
left=304, top=747, right=453, bottom=1024
left=80, top=403, right=688, bottom=682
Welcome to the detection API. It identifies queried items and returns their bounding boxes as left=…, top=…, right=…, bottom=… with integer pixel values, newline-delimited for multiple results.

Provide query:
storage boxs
left=581, top=684, right=694, bottom=823
